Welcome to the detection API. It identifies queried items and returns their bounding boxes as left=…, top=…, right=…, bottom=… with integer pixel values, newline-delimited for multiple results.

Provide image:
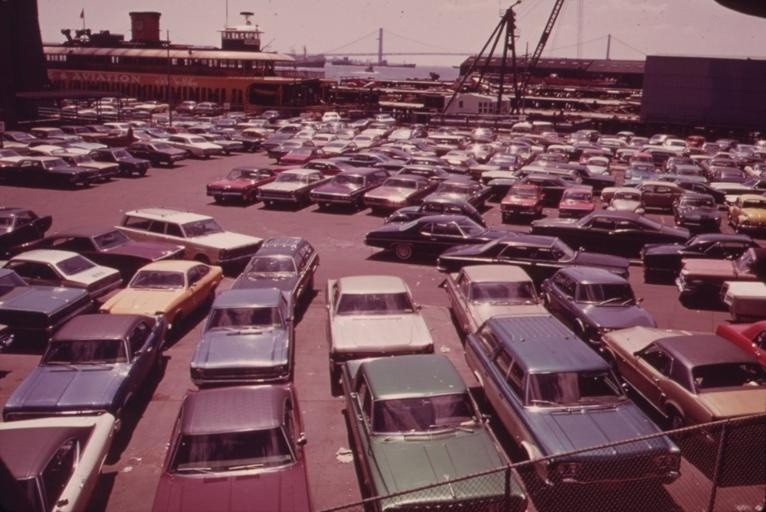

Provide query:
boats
left=41, top=8, right=645, bottom=119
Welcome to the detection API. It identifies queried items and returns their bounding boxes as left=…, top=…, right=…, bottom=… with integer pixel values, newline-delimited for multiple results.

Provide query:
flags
left=80, top=12, right=83, bottom=19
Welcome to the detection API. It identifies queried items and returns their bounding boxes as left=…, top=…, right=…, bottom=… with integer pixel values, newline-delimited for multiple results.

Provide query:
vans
left=230, top=236, right=320, bottom=312
left=465, top=312, right=682, bottom=490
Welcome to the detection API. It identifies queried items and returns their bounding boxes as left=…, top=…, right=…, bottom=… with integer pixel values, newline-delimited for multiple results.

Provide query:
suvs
left=0, top=98, right=766, bottom=234
left=113, top=206, right=264, bottom=266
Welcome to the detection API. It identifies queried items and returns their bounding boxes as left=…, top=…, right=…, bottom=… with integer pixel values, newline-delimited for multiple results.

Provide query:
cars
left=8, top=225, right=185, bottom=289
left=716, top=320, right=766, bottom=370
left=151, top=382, right=312, bottom=512
left=326, top=274, right=434, bottom=380
left=2, top=311, right=167, bottom=432
left=640, top=233, right=764, bottom=280
left=465, top=312, right=682, bottom=490
left=0, top=267, right=96, bottom=346
left=0, top=249, right=123, bottom=301
left=446, top=265, right=553, bottom=339
left=339, top=353, right=529, bottom=511
left=363, top=215, right=526, bottom=261
left=0, top=409, right=115, bottom=511
left=190, top=288, right=294, bottom=388
left=98, top=259, right=224, bottom=339
left=720, top=281, right=766, bottom=323
left=530, top=210, right=690, bottom=264
left=0, top=208, right=52, bottom=260
left=230, top=236, right=320, bottom=312
left=436, top=235, right=630, bottom=280
left=541, top=266, right=657, bottom=346
left=675, top=247, right=766, bottom=297
left=600, top=326, right=766, bottom=450
